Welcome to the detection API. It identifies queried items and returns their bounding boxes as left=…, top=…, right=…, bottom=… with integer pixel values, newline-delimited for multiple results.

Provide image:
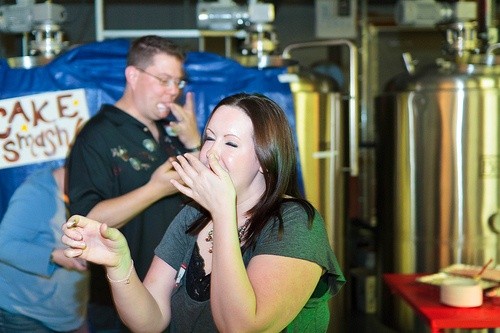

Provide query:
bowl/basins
left=437, top=277, right=484, bottom=307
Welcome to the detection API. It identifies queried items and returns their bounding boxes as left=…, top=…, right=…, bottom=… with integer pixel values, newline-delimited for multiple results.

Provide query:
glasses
left=135, top=67, right=186, bottom=90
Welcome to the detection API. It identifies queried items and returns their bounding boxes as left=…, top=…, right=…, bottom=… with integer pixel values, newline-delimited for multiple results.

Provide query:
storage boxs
left=352, top=268, right=380, bottom=314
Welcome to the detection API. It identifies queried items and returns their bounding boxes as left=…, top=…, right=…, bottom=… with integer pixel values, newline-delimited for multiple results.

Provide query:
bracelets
left=185, top=147, right=201, bottom=152
left=107, top=259, right=133, bottom=283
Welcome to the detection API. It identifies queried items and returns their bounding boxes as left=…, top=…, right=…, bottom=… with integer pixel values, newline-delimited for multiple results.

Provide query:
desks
left=384, top=272, right=500, bottom=333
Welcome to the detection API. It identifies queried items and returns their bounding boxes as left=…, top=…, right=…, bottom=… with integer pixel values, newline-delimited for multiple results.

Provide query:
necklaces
left=205, top=225, right=246, bottom=253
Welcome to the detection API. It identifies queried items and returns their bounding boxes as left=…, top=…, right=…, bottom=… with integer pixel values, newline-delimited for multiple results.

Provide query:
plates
left=414, top=263, right=500, bottom=297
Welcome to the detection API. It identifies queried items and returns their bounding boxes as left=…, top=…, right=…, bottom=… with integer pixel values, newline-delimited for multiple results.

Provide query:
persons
left=0, top=166, right=90, bottom=333
left=68, top=35, right=201, bottom=333
left=62, top=92, right=346, bottom=333
